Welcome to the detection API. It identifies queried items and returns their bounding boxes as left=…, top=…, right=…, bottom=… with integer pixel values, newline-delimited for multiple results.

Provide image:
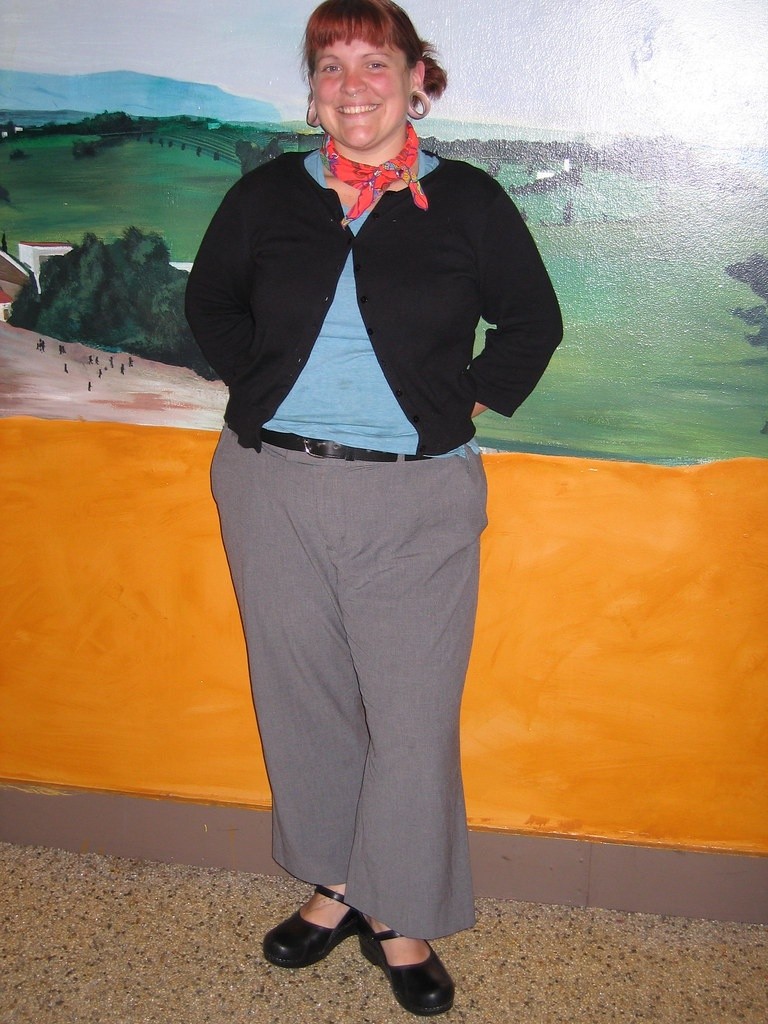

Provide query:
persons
left=187, top=0, right=562, bottom=1019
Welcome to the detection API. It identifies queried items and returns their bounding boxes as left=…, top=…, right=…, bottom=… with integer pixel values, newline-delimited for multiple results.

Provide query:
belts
left=261, top=428, right=431, bottom=461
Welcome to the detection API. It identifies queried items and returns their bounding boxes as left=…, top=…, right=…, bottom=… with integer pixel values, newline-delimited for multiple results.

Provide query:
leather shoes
left=263, top=886, right=365, bottom=968
left=357, top=920, right=457, bottom=1015
